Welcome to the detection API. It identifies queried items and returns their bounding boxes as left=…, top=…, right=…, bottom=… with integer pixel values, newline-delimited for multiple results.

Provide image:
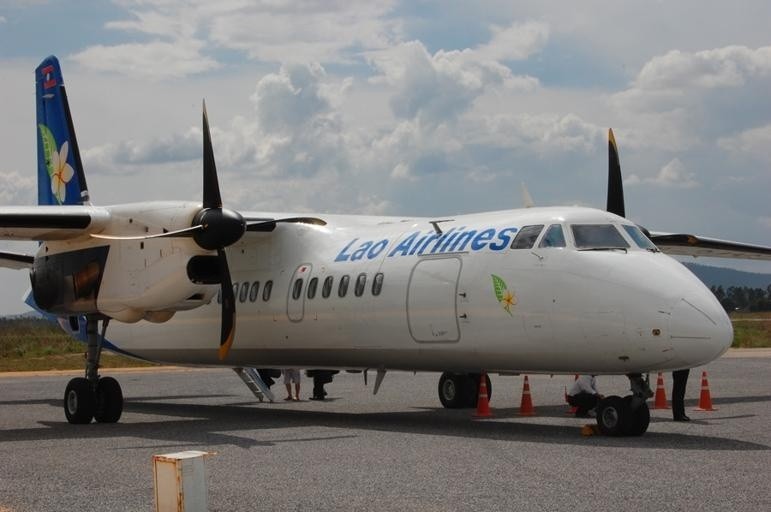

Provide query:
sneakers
left=674, top=416, right=690, bottom=420
left=283, top=391, right=328, bottom=401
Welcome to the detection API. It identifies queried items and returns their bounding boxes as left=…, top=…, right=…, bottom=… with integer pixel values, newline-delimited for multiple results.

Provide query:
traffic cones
left=692, top=370, right=719, bottom=414
left=649, top=372, right=672, bottom=412
left=513, top=374, right=538, bottom=419
left=472, top=368, right=497, bottom=419
left=565, top=373, right=581, bottom=414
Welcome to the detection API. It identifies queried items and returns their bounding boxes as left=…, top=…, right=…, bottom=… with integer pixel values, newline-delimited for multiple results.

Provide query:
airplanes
left=0, top=53, right=771, bottom=442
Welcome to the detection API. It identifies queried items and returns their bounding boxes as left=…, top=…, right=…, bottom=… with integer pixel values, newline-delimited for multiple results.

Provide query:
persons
left=282, top=367, right=302, bottom=401
left=566, top=373, right=608, bottom=418
left=306, top=368, right=342, bottom=402
